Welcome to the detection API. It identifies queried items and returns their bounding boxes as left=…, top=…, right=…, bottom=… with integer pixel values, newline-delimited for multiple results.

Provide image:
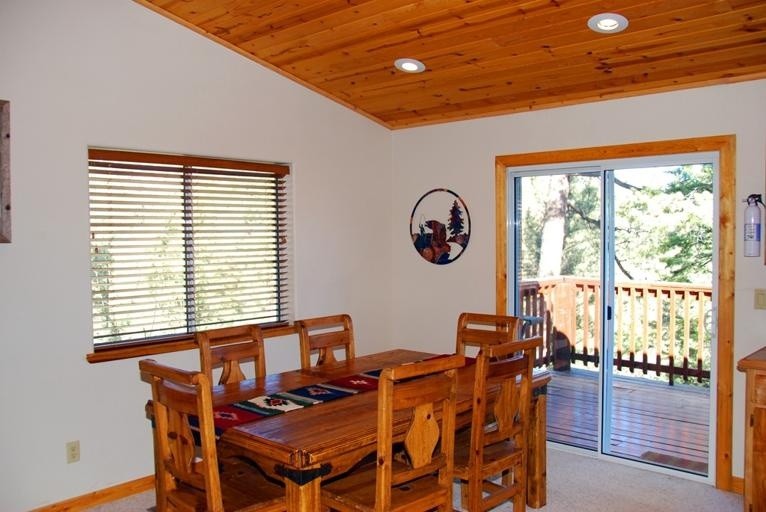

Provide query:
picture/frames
left=409, top=187, right=471, bottom=265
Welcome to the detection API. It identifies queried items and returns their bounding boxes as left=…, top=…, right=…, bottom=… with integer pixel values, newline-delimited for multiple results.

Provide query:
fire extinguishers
left=742, top=194, right=766, bottom=257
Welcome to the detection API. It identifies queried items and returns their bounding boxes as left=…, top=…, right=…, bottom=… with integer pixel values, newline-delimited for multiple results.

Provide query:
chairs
left=293, top=311, right=394, bottom=481
left=454, top=310, right=524, bottom=504
left=193, top=323, right=268, bottom=389
left=138, top=356, right=291, bottom=512
left=320, top=351, right=471, bottom=512
left=386, top=333, right=545, bottom=512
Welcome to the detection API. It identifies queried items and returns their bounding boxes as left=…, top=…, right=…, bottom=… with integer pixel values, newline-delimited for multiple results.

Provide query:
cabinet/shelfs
left=737, top=344, right=766, bottom=512
